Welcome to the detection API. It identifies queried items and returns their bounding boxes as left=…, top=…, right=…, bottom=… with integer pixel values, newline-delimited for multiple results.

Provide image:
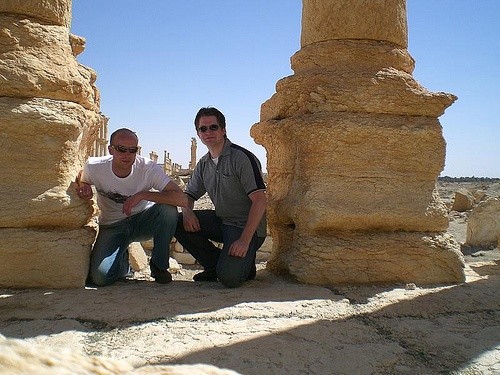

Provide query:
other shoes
left=193, top=271, right=215, bottom=281
left=151, top=270, right=173, bottom=283
left=247, top=261, right=256, bottom=280
left=126, top=261, right=134, bottom=277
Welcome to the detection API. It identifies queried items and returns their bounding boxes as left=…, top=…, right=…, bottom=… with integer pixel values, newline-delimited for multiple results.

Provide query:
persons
left=77, top=128, right=189, bottom=287
left=173, top=107, right=267, bottom=288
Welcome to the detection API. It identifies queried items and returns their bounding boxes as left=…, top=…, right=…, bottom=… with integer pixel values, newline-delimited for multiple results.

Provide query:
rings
left=80, top=190, right=82, bottom=193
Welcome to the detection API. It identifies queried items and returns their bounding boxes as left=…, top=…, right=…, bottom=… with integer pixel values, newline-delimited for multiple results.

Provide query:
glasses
left=111, top=144, right=138, bottom=153
left=197, top=124, right=219, bottom=132
